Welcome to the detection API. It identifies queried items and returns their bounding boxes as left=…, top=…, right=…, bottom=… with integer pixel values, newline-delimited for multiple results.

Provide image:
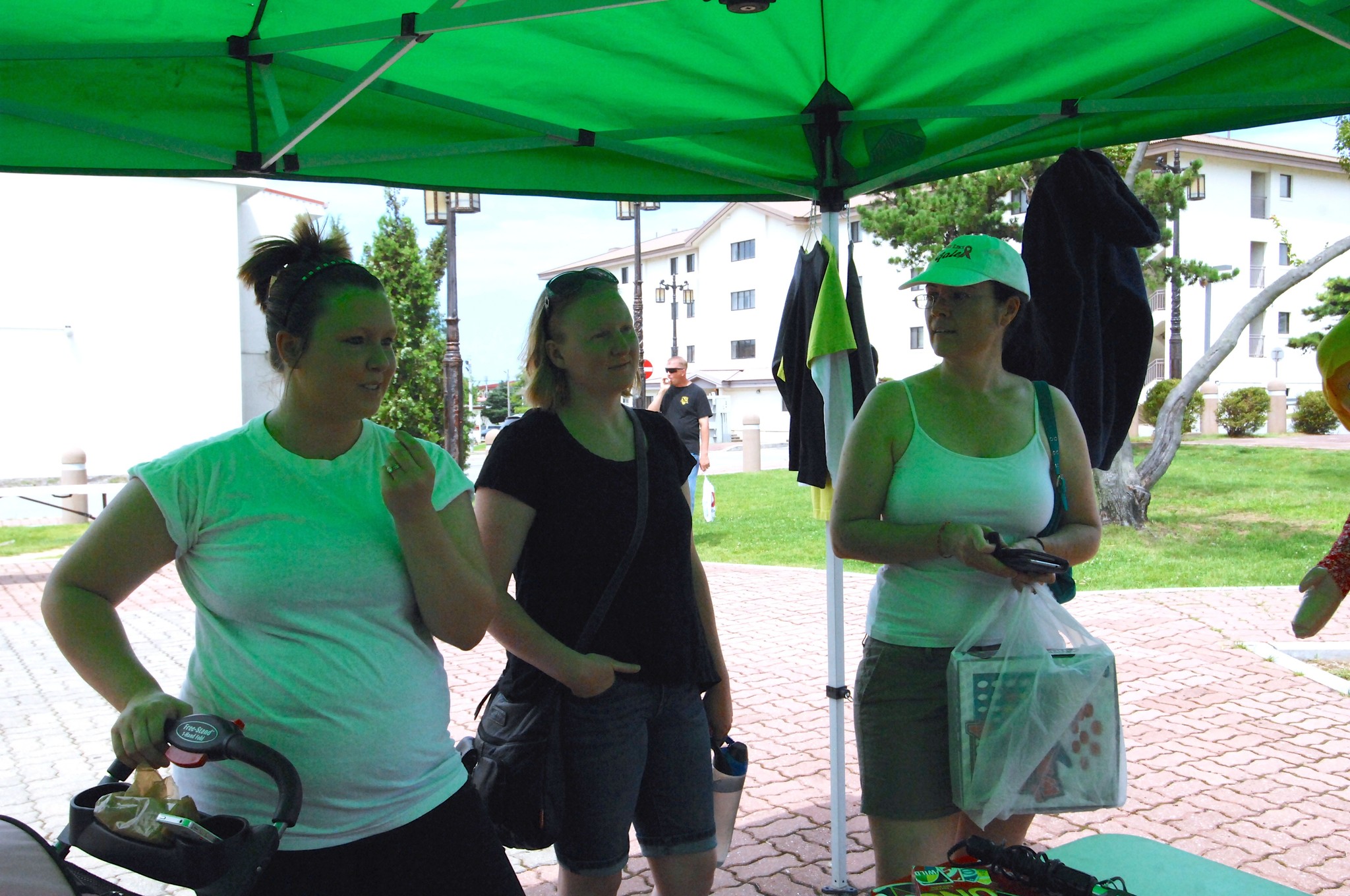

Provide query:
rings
left=387, top=463, right=400, bottom=473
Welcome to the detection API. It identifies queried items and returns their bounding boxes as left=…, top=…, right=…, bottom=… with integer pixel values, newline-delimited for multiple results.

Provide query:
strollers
left=0, top=712, right=307, bottom=896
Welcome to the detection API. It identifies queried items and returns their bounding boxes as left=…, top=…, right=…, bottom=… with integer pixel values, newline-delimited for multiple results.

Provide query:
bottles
left=711, top=734, right=749, bottom=868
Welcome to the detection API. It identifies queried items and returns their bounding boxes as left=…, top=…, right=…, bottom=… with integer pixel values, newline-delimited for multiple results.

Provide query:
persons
left=461, top=264, right=737, bottom=896
left=40, top=214, right=533, bottom=896
left=829, top=234, right=1103, bottom=888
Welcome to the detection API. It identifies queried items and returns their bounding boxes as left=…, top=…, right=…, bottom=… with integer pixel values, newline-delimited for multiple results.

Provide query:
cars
left=499, top=413, right=524, bottom=432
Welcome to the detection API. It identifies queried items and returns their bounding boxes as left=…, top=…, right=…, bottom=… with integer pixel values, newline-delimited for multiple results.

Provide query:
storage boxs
left=950, top=651, right=1119, bottom=811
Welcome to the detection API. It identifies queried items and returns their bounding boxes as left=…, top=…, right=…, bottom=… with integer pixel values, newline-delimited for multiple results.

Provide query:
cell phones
left=1000, top=557, right=1062, bottom=568
left=157, top=813, right=223, bottom=843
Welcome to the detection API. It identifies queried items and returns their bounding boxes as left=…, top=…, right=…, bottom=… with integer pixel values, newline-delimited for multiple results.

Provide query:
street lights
left=1153, top=149, right=1206, bottom=380
left=655, top=273, right=694, bottom=358
left=616, top=199, right=660, bottom=405
left=422, top=186, right=483, bottom=474
left=503, top=369, right=511, bottom=417
left=464, top=360, right=473, bottom=411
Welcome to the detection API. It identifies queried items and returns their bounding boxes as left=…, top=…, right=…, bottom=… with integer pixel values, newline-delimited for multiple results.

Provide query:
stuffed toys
left=1294, top=314, right=1349, bottom=640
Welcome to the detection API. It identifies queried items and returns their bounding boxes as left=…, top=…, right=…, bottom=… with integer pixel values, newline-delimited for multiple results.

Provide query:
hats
left=898, top=235, right=1031, bottom=304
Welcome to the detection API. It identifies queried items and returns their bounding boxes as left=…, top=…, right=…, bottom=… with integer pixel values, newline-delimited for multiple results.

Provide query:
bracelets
left=1026, top=536, right=1046, bottom=550
left=937, top=523, right=954, bottom=559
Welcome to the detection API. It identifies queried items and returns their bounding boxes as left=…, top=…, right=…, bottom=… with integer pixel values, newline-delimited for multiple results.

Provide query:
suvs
left=481, top=425, right=502, bottom=437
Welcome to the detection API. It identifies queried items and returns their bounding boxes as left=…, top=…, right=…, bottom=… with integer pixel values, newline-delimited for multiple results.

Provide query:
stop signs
left=641, top=359, right=654, bottom=380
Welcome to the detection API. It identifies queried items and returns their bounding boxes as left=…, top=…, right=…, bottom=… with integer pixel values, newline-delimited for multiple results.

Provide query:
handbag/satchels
left=944, top=579, right=1127, bottom=831
left=703, top=474, right=715, bottom=524
left=456, top=676, right=556, bottom=851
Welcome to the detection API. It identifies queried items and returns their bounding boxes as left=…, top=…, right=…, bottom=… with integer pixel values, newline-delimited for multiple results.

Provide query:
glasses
left=664, top=368, right=685, bottom=373
left=912, top=291, right=1010, bottom=309
left=545, top=267, right=619, bottom=325
